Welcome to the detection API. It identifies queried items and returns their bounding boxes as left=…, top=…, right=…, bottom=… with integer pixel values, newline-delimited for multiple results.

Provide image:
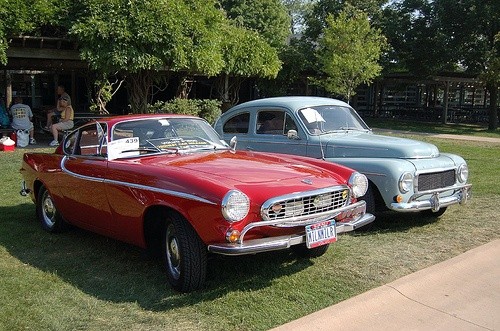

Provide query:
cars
left=18, top=113, right=377, bottom=293
left=179, top=96, right=474, bottom=224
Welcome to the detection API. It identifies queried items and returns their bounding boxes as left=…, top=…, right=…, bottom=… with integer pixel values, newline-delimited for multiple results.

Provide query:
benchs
left=62, top=130, right=90, bottom=138
left=0, top=126, right=16, bottom=135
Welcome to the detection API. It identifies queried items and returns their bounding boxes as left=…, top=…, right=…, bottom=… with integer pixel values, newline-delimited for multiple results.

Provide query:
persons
left=45, top=86, right=71, bottom=128
left=11, top=98, right=36, bottom=144
left=49, top=96, right=74, bottom=146
left=0, top=92, right=2, bottom=104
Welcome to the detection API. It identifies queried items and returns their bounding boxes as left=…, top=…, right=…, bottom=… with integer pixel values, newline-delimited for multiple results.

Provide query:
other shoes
left=29, top=138, right=37, bottom=145
left=43, top=125, right=53, bottom=132
left=48, top=140, right=60, bottom=146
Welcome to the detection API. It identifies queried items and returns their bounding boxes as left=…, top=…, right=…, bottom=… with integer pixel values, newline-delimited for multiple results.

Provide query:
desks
left=72, top=113, right=112, bottom=128
